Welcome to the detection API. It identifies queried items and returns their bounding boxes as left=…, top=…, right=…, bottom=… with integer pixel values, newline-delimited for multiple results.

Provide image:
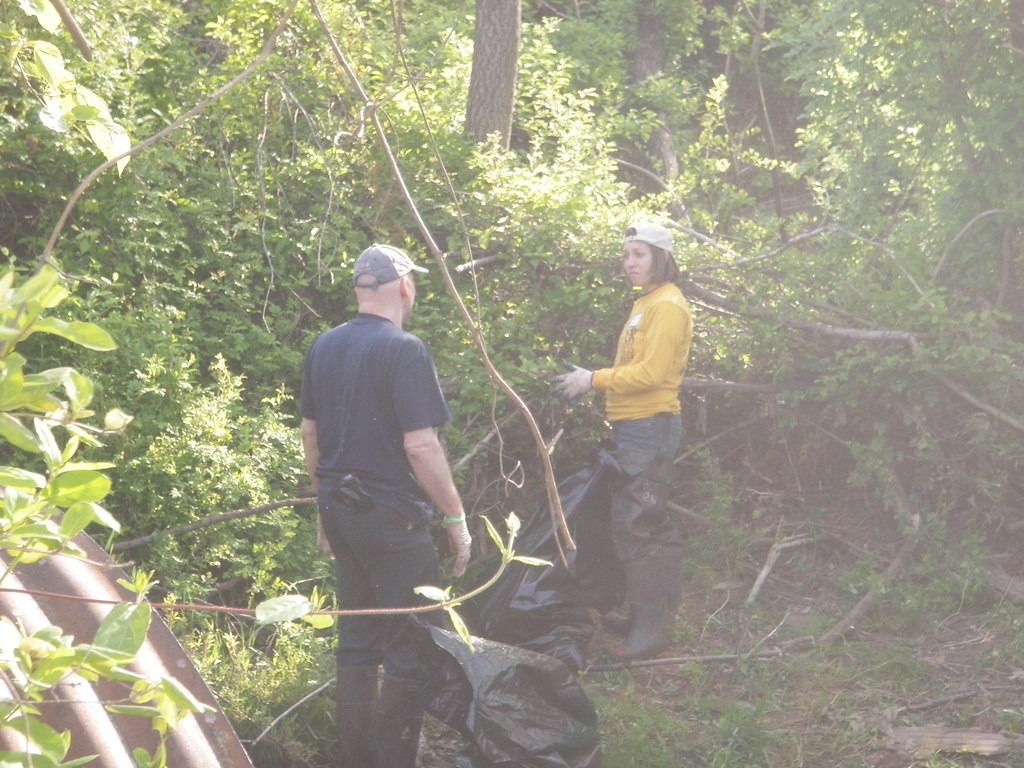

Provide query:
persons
left=300, top=243, right=473, bottom=768
left=550, top=222, right=693, bottom=660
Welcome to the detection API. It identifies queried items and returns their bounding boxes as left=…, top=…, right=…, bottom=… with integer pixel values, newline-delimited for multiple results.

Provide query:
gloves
left=550, top=359, right=594, bottom=401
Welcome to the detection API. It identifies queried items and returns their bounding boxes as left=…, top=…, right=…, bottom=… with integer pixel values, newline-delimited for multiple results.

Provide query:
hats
left=353, top=243, right=429, bottom=287
left=624, top=222, right=681, bottom=275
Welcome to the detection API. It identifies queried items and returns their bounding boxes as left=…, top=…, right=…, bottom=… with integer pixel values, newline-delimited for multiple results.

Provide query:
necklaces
left=639, top=283, right=667, bottom=296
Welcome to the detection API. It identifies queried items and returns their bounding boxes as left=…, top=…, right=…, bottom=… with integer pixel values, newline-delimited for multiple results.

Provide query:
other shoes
left=604, top=598, right=636, bottom=628
left=606, top=639, right=667, bottom=662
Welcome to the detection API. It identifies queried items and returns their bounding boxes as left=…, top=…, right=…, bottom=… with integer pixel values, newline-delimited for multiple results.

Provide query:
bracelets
left=590, top=371, right=595, bottom=389
left=441, top=512, right=465, bottom=529
